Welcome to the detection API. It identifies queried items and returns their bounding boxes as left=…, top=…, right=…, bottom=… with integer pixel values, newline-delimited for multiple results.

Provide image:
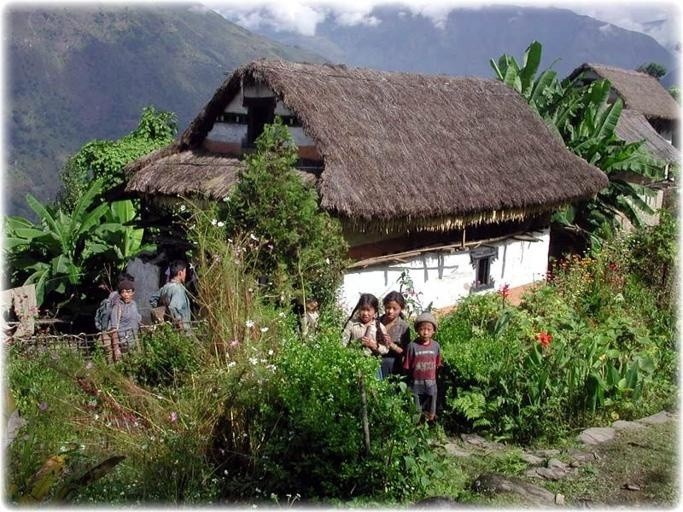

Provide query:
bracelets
left=392, top=344, right=397, bottom=351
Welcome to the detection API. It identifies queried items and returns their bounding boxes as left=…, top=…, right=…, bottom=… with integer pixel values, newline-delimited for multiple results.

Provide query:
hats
left=414, top=313, right=438, bottom=334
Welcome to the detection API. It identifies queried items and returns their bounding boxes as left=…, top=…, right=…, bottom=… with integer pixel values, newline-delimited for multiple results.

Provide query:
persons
left=98, top=260, right=192, bottom=366
left=376, top=291, right=411, bottom=384
left=403, top=311, right=445, bottom=422
left=339, top=294, right=389, bottom=379
left=300, top=298, right=321, bottom=341
